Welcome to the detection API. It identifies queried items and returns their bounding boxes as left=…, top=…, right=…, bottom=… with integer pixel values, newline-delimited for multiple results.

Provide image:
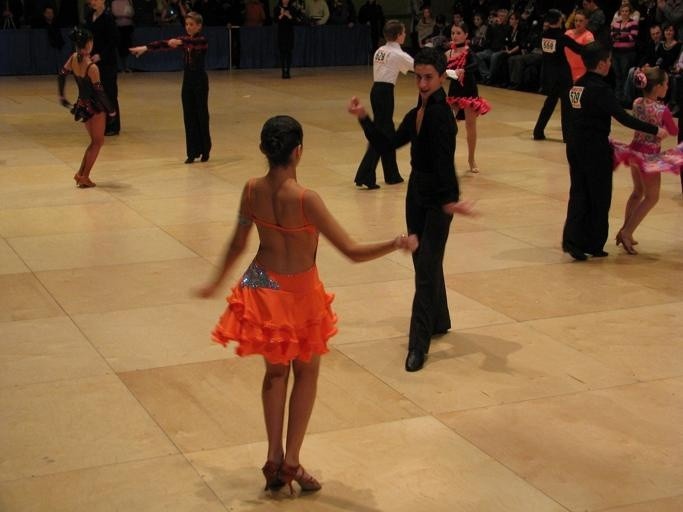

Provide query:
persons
left=127, top=10, right=212, bottom=164
left=73, top=0, right=122, bottom=137
left=1, top=1, right=387, bottom=81
left=345, top=47, right=485, bottom=374
left=54, top=25, right=118, bottom=189
left=533, top=8, right=594, bottom=140
left=441, top=21, right=493, bottom=174
left=608, top=63, right=682, bottom=256
left=352, top=18, right=417, bottom=191
left=187, top=114, right=421, bottom=497
left=404, top=1, right=683, bottom=112
left=558, top=39, right=669, bottom=261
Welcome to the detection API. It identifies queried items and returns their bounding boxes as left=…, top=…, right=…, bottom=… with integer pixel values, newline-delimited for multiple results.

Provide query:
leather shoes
left=406, top=349, right=423, bottom=370
left=201, top=151, right=208, bottom=161
left=185, top=153, right=199, bottom=163
left=563, top=243, right=608, bottom=260
left=468, top=159, right=479, bottom=172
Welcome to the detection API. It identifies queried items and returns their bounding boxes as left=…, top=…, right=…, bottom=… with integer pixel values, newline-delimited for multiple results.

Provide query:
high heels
left=354, top=176, right=380, bottom=189
left=75, top=173, right=96, bottom=187
left=615, top=229, right=638, bottom=254
left=263, top=459, right=321, bottom=494
